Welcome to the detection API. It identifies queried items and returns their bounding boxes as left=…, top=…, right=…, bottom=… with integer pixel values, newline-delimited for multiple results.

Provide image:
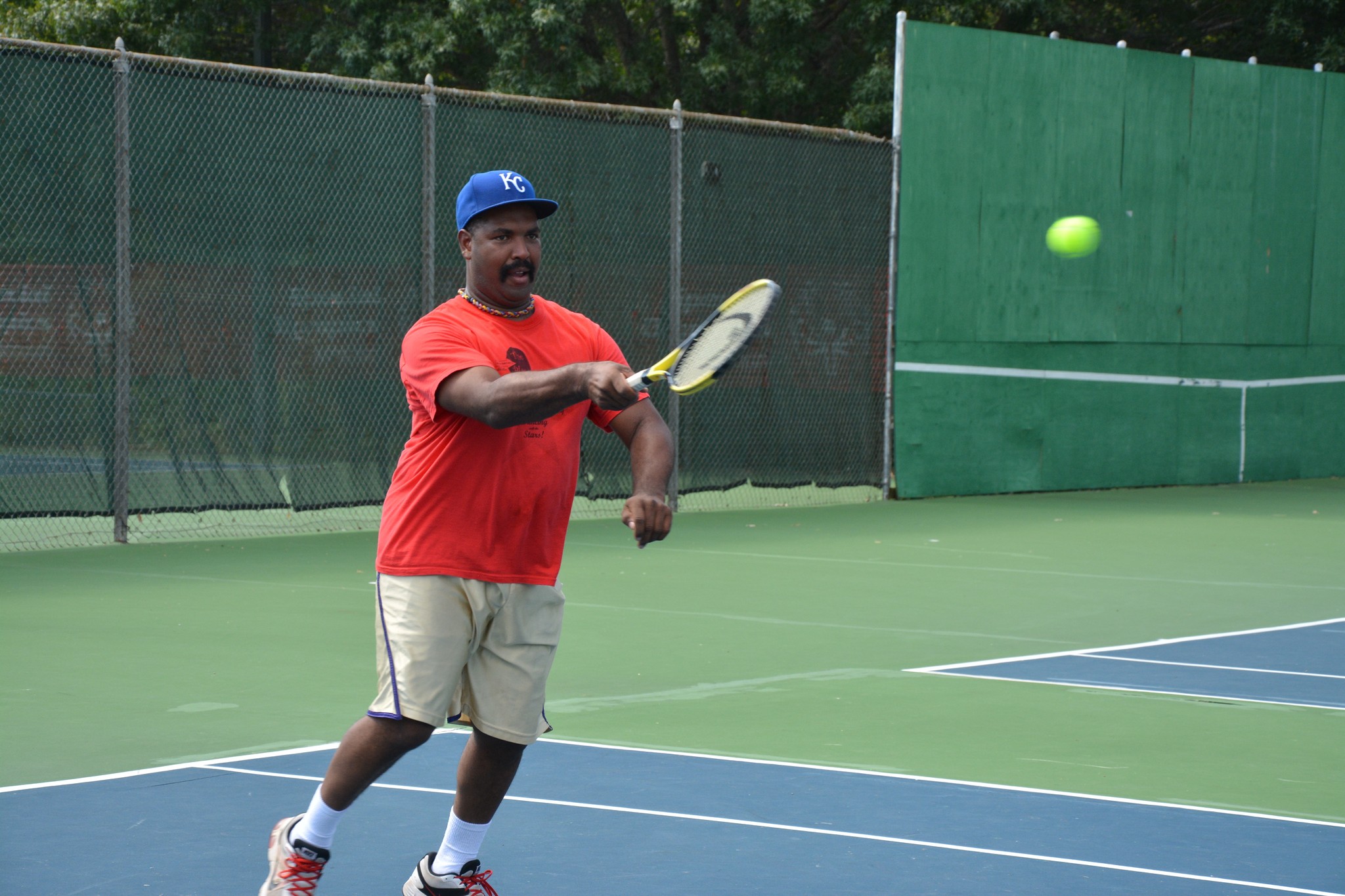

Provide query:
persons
left=259, top=170, right=675, bottom=896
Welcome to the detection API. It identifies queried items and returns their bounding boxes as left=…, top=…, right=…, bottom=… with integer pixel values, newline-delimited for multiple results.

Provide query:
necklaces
left=458, top=288, right=535, bottom=318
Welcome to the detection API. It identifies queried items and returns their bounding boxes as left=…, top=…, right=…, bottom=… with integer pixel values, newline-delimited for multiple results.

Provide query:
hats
left=456, top=172, right=558, bottom=231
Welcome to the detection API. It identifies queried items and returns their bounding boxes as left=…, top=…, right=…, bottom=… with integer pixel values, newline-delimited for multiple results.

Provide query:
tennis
left=1046, top=216, right=1100, bottom=259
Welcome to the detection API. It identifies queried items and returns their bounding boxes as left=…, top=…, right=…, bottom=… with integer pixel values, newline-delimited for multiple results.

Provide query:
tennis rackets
left=624, top=278, right=782, bottom=396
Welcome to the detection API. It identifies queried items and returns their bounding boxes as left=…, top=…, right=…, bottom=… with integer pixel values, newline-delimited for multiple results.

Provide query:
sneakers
left=402, top=852, right=497, bottom=896
left=258, top=814, right=330, bottom=896
